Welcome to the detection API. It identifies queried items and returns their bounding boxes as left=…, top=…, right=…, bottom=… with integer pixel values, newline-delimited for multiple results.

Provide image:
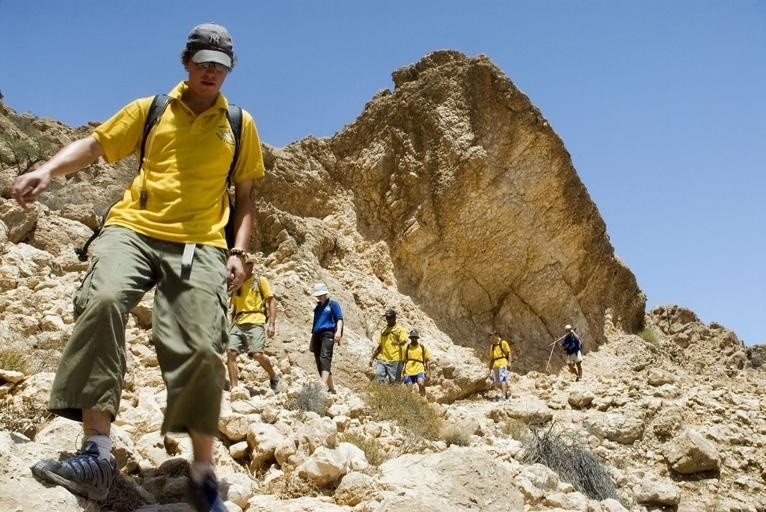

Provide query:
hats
left=312, top=283, right=328, bottom=297
left=408, top=330, right=420, bottom=339
left=186, top=22, right=235, bottom=70
left=245, top=252, right=256, bottom=265
left=382, top=309, right=396, bottom=317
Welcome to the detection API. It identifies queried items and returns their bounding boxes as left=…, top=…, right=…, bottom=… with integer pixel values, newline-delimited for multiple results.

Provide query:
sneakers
left=33, top=440, right=117, bottom=501
left=270, top=375, right=282, bottom=393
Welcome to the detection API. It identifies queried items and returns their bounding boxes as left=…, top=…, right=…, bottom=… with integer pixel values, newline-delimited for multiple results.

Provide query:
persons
left=369, top=310, right=408, bottom=385
left=489, top=332, right=512, bottom=399
left=226, top=253, right=280, bottom=390
left=555, top=324, right=582, bottom=382
left=13, top=22, right=266, bottom=512
left=401, top=330, right=430, bottom=398
left=311, top=283, right=343, bottom=394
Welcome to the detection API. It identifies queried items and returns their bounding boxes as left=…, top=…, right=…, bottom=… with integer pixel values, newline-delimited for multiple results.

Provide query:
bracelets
left=270, top=321, right=275, bottom=324
left=230, top=248, right=249, bottom=261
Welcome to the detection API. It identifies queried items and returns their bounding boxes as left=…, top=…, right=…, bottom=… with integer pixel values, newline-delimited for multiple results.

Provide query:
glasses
left=197, top=63, right=227, bottom=71
